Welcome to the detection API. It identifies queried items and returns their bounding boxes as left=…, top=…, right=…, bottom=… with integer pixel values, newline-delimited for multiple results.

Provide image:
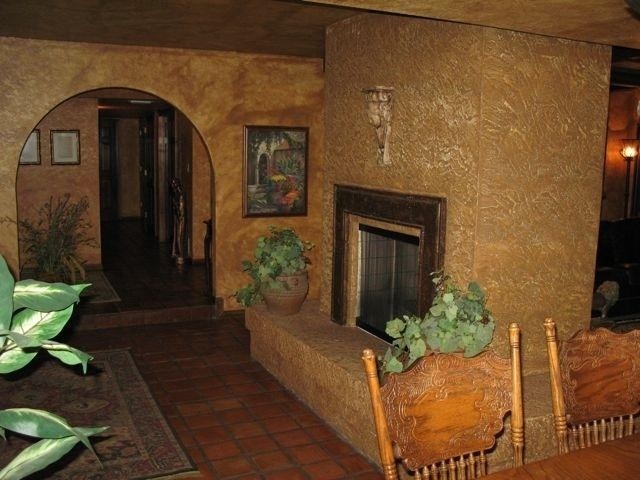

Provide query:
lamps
left=619, top=138, right=640, bottom=219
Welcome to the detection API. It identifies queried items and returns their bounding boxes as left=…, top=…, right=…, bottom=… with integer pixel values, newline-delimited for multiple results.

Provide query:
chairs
left=361, top=321, right=526, bottom=479
left=541, top=318, right=640, bottom=456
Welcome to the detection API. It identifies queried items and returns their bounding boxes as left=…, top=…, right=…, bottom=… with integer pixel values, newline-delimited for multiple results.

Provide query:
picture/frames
left=19, top=128, right=41, bottom=165
left=50, top=130, right=80, bottom=165
left=243, top=125, right=309, bottom=218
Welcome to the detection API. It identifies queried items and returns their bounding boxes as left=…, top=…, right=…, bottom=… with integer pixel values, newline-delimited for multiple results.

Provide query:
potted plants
left=229, top=224, right=318, bottom=316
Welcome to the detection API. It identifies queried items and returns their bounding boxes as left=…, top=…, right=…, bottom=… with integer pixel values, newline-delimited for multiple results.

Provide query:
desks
left=475, top=432, right=639, bottom=479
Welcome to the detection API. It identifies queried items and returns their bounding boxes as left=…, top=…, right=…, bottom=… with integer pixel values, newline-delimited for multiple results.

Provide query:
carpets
left=0, top=345, right=203, bottom=478
left=21, top=270, right=121, bottom=303
